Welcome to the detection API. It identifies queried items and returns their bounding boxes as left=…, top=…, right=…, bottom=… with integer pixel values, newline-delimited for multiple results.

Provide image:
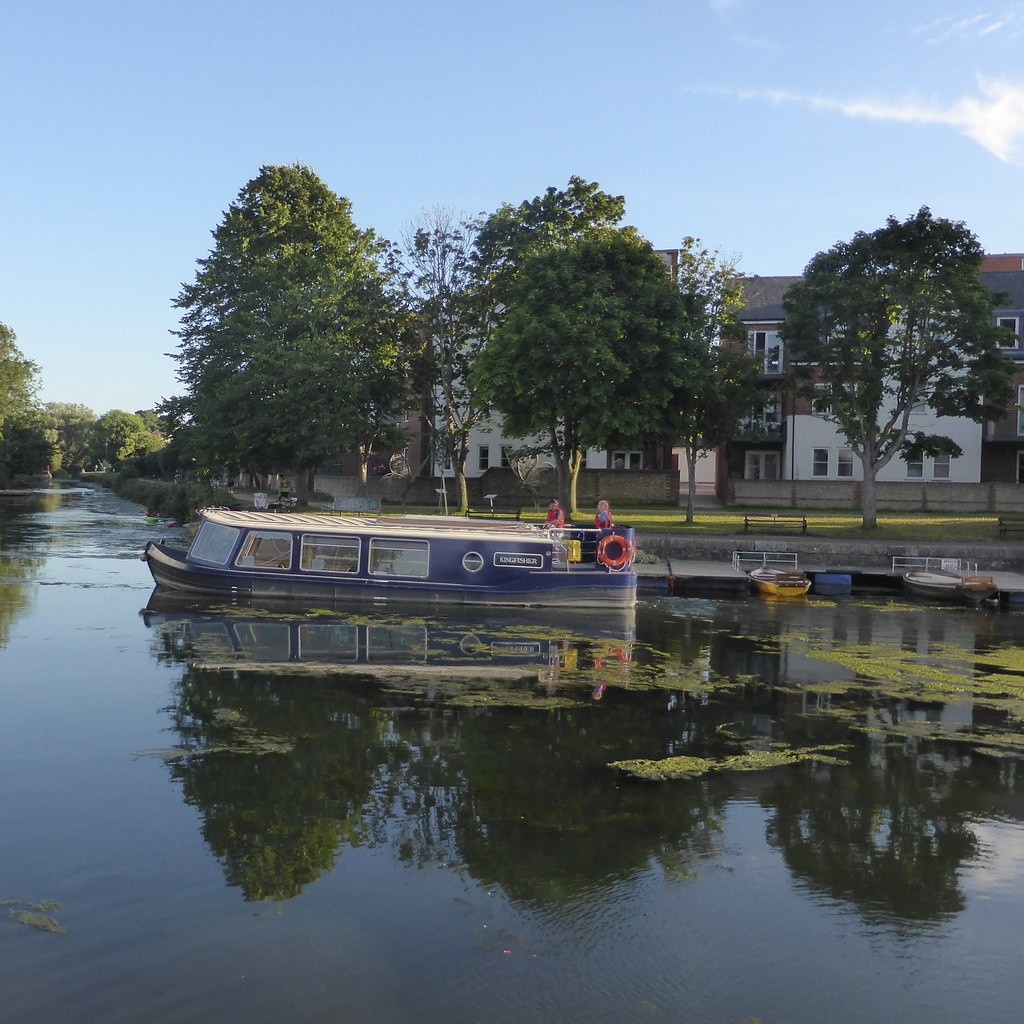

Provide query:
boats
left=138, top=585, right=635, bottom=699
left=144, top=505, right=638, bottom=610
left=145, top=515, right=164, bottom=524
left=905, top=569, right=998, bottom=605
left=748, top=566, right=812, bottom=597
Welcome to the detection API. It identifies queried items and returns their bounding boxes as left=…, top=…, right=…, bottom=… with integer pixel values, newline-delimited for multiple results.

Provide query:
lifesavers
left=596, top=535, right=630, bottom=566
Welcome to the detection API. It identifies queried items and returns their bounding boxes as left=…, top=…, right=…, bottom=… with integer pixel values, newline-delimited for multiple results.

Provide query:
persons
left=594, top=499, right=614, bottom=529
left=543, top=500, right=563, bottom=562
left=227, top=479, right=234, bottom=494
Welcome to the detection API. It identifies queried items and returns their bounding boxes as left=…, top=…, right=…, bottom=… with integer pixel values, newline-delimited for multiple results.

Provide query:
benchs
left=998, top=517, right=1024, bottom=541
left=321, top=496, right=381, bottom=518
left=744, top=512, right=807, bottom=536
left=465, top=505, right=522, bottom=521
left=756, top=573, right=800, bottom=580
left=279, top=496, right=299, bottom=507
